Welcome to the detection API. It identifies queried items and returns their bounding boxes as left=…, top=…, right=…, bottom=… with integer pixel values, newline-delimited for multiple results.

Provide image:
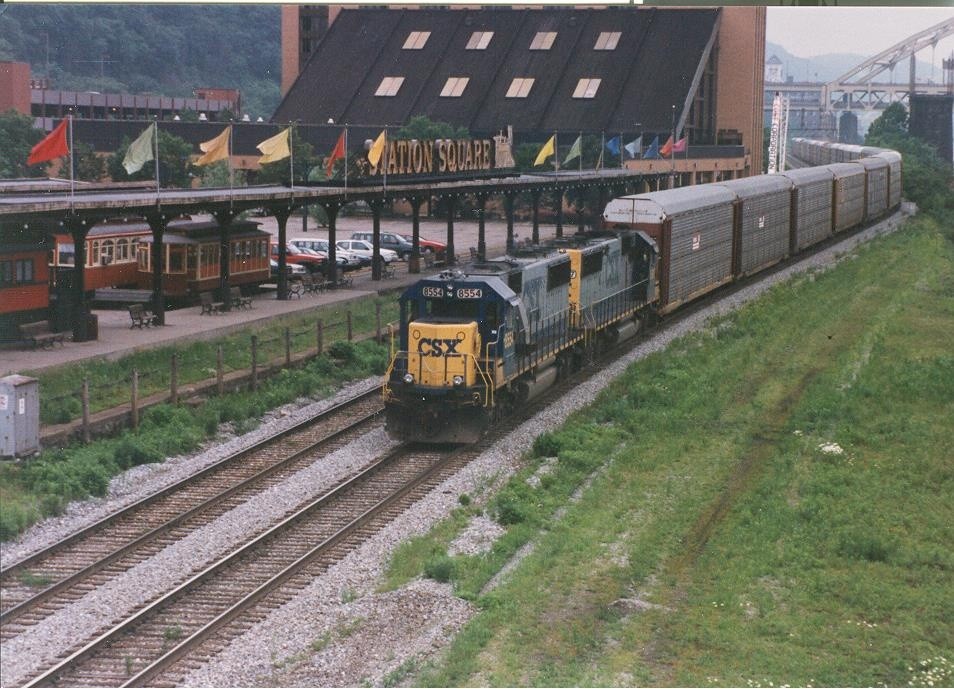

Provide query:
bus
left=0, top=218, right=271, bottom=326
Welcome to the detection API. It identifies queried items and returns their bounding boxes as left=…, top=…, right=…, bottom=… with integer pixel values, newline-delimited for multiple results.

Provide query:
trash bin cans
left=72, top=314, right=98, bottom=341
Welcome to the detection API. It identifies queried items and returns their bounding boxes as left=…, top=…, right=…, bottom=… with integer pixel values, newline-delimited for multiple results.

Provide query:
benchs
left=286, top=247, right=482, bottom=301
left=231, top=287, right=251, bottom=309
left=128, top=304, right=158, bottom=328
left=198, top=291, right=225, bottom=317
left=17, top=320, right=66, bottom=349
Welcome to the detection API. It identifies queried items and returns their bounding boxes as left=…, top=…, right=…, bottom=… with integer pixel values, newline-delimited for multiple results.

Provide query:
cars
left=335, top=239, right=398, bottom=267
left=268, top=239, right=346, bottom=276
left=384, top=233, right=447, bottom=255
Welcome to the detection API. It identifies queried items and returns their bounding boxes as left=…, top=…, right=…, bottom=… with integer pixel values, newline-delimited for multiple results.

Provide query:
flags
left=368, top=130, right=385, bottom=168
left=198, top=125, right=231, bottom=167
left=660, top=135, right=674, bottom=157
left=123, top=122, right=155, bottom=176
left=673, top=136, right=686, bottom=152
left=534, top=135, right=555, bottom=167
left=26, top=117, right=70, bottom=167
left=561, top=135, right=581, bottom=167
left=605, top=134, right=621, bottom=156
left=326, top=130, right=345, bottom=177
left=643, top=136, right=659, bottom=159
left=625, top=136, right=642, bottom=160
left=255, top=128, right=291, bottom=165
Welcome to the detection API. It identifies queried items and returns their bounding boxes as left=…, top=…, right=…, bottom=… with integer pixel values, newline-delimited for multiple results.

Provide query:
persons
left=425, top=249, right=435, bottom=267
left=511, top=232, right=532, bottom=249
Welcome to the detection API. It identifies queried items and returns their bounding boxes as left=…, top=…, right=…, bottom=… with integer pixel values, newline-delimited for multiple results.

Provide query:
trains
left=381, top=135, right=903, bottom=447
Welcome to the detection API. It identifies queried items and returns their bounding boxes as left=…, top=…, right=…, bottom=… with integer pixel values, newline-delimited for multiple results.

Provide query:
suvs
left=349, top=232, right=413, bottom=262
left=288, top=237, right=361, bottom=269
